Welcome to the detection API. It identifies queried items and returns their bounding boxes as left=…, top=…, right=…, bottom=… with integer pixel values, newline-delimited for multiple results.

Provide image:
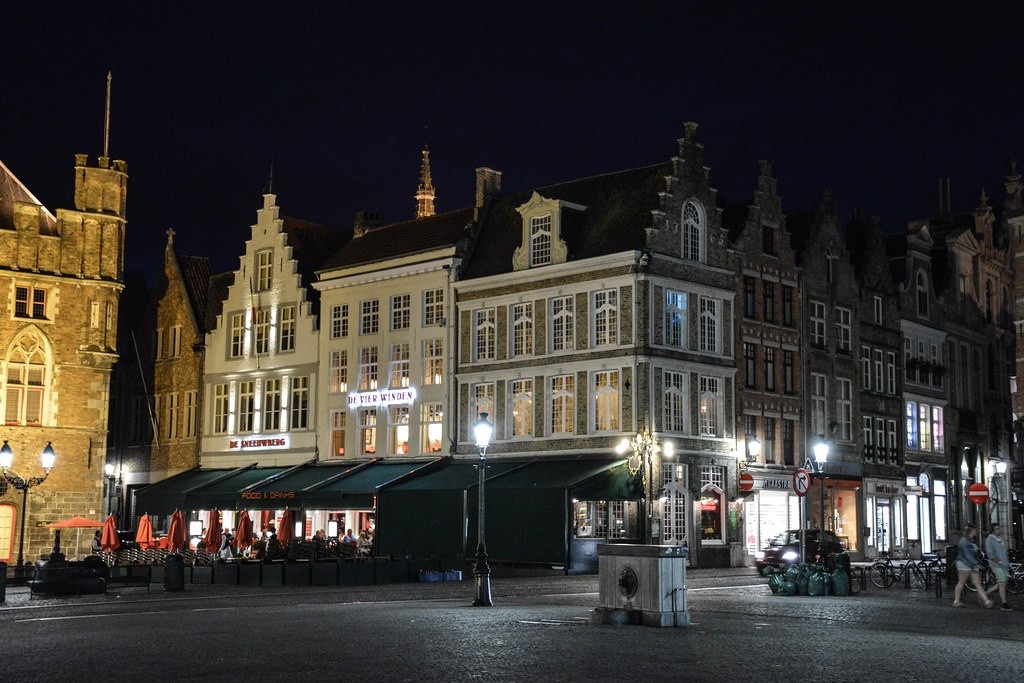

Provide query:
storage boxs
left=420, top=571, right=461, bottom=582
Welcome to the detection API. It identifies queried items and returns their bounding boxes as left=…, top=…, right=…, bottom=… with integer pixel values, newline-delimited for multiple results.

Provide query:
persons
left=91, top=531, right=103, bottom=556
left=197, top=527, right=236, bottom=549
left=986, top=523, right=1013, bottom=611
left=312, top=529, right=326, bottom=540
left=339, top=529, right=375, bottom=549
left=952, top=524, right=996, bottom=608
left=253, top=527, right=277, bottom=541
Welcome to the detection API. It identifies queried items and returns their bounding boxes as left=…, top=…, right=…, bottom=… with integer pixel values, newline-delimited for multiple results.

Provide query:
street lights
left=813, top=442, right=829, bottom=565
left=105, top=463, right=114, bottom=517
left=470, top=413, right=492, bottom=606
left=1, top=440, right=56, bottom=585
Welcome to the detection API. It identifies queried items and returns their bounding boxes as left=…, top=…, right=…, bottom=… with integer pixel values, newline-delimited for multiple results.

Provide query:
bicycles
left=870, top=541, right=928, bottom=591
left=828, top=547, right=861, bottom=593
left=920, top=544, right=1024, bottom=594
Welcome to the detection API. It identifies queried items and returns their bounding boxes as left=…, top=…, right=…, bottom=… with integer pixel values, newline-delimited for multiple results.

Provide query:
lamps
left=742, top=436, right=762, bottom=471
left=992, top=460, right=1008, bottom=480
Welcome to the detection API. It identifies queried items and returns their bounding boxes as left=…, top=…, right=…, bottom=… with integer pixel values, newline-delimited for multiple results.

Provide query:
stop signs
left=968, top=483, right=989, bottom=504
left=739, top=474, right=754, bottom=490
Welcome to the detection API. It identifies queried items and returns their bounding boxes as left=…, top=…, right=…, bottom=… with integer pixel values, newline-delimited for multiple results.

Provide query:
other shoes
left=1000, top=603, right=1013, bottom=610
left=985, top=601, right=994, bottom=608
left=953, top=601, right=966, bottom=608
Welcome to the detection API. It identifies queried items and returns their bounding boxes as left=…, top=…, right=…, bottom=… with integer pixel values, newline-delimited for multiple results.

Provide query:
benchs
left=25, top=562, right=151, bottom=601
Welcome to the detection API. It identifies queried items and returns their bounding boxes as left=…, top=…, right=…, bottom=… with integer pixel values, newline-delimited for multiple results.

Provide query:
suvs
left=755, top=530, right=843, bottom=577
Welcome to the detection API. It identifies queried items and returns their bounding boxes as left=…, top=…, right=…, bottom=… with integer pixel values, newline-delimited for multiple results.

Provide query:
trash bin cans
left=163, top=555, right=186, bottom=591
left=811, top=553, right=851, bottom=594
left=945, top=546, right=959, bottom=584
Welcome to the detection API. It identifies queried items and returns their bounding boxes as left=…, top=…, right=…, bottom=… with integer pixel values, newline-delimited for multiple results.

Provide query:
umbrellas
left=167, top=507, right=186, bottom=554
left=102, top=511, right=120, bottom=566
left=44, top=515, right=106, bottom=557
left=204, top=507, right=222, bottom=560
left=135, top=511, right=152, bottom=550
left=235, top=511, right=253, bottom=557
left=276, top=506, right=294, bottom=546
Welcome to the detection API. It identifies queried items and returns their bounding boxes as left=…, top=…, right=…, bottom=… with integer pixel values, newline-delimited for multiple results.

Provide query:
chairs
left=93, top=537, right=356, bottom=566
